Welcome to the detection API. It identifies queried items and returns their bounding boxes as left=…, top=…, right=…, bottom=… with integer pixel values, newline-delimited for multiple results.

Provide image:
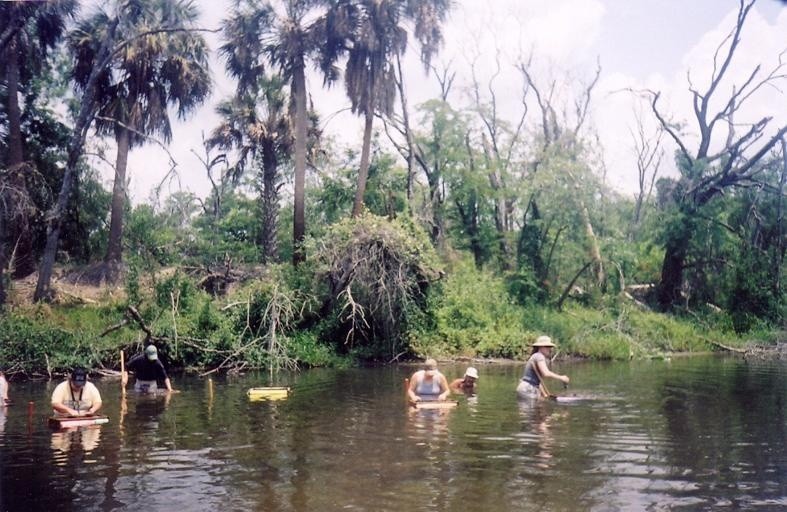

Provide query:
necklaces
left=68, top=380, right=85, bottom=411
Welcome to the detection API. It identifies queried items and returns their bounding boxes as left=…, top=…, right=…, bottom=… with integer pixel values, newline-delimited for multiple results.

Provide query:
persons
left=49, top=366, right=104, bottom=418
left=515, top=334, right=571, bottom=401
left=406, top=359, right=450, bottom=401
left=449, top=366, right=480, bottom=396
left=0, top=368, right=10, bottom=406
left=119, top=345, right=173, bottom=394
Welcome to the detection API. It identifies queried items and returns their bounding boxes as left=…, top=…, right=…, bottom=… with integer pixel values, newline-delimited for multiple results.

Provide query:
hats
left=72, top=369, right=86, bottom=388
left=466, top=367, right=479, bottom=379
left=531, top=336, right=555, bottom=348
left=424, top=359, right=437, bottom=376
left=146, top=345, right=159, bottom=361
